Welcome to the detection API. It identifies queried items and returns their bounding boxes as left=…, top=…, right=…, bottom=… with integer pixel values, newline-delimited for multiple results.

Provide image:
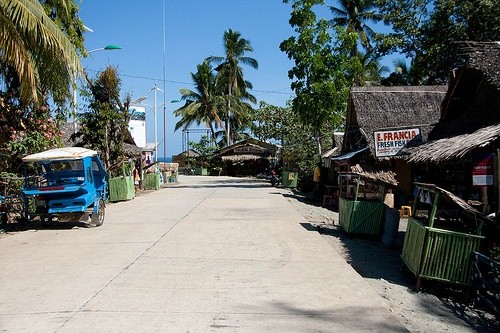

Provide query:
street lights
left=73, top=43, right=123, bottom=144
left=154, top=99, right=183, bottom=171
